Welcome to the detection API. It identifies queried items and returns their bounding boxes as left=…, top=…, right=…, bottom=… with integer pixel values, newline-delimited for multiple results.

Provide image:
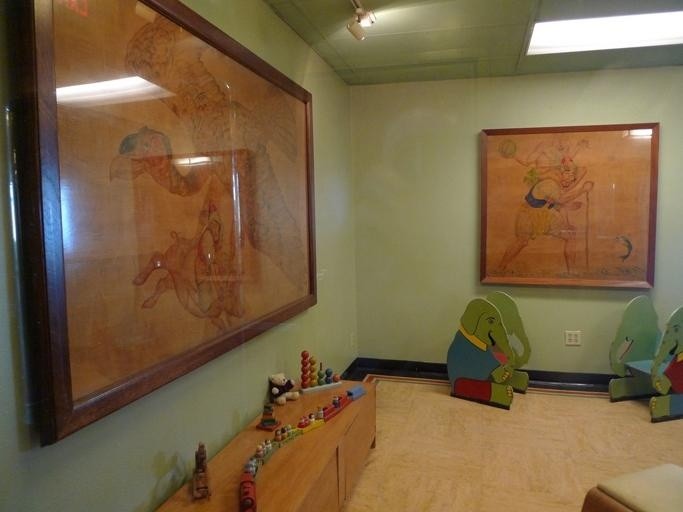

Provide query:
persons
left=494, top=144, right=595, bottom=278
left=504, top=132, right=589, bottom=194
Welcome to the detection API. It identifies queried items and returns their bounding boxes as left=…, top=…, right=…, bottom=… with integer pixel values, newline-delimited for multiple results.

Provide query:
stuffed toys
left=268, top=372, right=299, bottom=406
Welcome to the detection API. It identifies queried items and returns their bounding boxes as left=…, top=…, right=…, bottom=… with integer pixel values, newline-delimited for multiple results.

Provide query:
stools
left=605, top=295, right=682, bottom=422
left=581, top=462, right=683, bottom=511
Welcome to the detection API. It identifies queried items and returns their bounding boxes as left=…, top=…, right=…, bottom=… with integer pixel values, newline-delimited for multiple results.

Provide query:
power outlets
left=565, top=331, right=581, bottom=346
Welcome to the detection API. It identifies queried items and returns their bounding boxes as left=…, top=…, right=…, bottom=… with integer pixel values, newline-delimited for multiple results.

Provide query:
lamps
left=347, top=7, right=368, bottom=41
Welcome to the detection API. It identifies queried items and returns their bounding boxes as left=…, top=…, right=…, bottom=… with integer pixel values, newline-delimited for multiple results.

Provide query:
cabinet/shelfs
left=480, top=122, right=661, bottom=290
left=153, top=380, right=376, bottom=510
left=5, top=0, right=317, bottom=448
